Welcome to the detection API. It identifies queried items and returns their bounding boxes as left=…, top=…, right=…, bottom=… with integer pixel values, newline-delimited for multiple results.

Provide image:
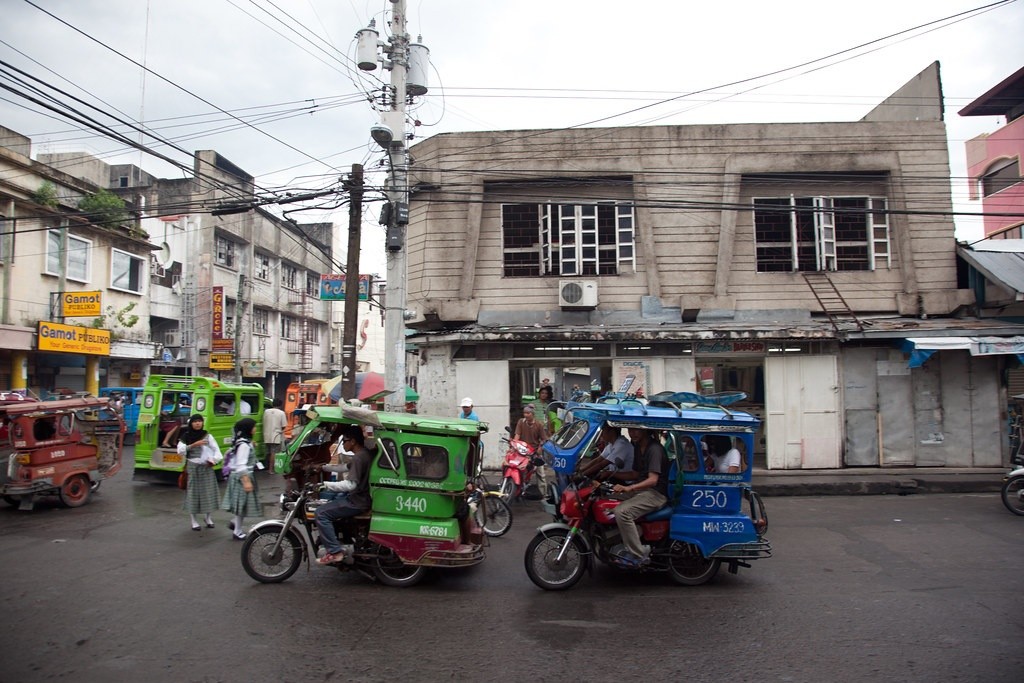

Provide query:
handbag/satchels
left=177, top=473, right=187, bottom=489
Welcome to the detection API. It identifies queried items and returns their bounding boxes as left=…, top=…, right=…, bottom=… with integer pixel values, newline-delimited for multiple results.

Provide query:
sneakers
left=316, top=552, right=343, bottom=564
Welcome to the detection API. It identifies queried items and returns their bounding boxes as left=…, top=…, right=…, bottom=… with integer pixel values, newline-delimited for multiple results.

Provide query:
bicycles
left=474, top=472, right=513, bottom=538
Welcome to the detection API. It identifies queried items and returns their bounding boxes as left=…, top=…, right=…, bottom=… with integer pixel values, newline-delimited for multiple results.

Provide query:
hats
left=461, top=397, right=474, bottom=407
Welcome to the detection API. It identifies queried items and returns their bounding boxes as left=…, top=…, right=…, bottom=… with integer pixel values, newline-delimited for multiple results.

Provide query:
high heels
left=227, top=521, right=235, bottom=530
left=233, top=533, right=246, bottom=539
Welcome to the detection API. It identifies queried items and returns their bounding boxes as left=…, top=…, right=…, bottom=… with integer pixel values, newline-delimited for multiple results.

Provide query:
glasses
left=342, top=439, right=351, bottom=445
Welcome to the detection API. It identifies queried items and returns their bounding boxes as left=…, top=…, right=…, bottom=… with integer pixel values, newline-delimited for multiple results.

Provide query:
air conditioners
left=558, top=279, right=600, bottom=310
left=164, top=332, right=182, bottom=348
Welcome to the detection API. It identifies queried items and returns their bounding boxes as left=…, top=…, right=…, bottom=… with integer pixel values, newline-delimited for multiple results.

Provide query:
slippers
left=162, top=444, right=171, bottom=448
left=172, top=445, right=178, bottom=448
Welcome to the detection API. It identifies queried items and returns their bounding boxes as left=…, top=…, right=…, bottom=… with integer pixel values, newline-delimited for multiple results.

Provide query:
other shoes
left=191, top=523, right=201, bottom=530
left=204, top=519, right=215, bottom=528
left=614, top=557, right=642, bottom=568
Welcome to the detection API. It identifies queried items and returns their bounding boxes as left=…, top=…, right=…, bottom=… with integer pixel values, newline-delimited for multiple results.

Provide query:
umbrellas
left=321, top=370, right=420, bottom=403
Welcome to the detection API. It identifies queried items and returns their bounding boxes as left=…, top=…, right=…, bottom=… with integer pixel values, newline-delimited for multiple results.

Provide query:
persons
left=222, top=418, right=264, bottom=540
left=227, top=398, right=251, bottom=414
left=177, top=414, right=224, bottom=530
left=459, top=397, right=483, bottom=448
left=160, top=393, right=180, bottom=448
left=313, top=426, right=374, bottom=565
left=263, top=398, right=287, bottom=475
left=102, top=390, right=144, bottom=419
left=513, top=378, right=741, bottom=568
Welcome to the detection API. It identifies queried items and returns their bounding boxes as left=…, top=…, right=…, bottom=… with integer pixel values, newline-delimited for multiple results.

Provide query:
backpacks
left=222, top=442, right=249, bottom=480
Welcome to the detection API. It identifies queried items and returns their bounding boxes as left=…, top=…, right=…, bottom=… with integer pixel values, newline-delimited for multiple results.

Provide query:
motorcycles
left=497, top=425, right=546, bottom=514
left=98, top=387, right=191, bottom=447
left=284, top=383, right=337, bottom=441
left=132, top=374, right=265, bottom=489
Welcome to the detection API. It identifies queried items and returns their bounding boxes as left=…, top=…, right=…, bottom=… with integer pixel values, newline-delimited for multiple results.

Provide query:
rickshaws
left=1001, top=394, right=1024, bottom=516
left=0, top=389, right=124, bottom=512
left=240, top=404, right=486, bottom=587
left=524, top=390, right=773, bottom=593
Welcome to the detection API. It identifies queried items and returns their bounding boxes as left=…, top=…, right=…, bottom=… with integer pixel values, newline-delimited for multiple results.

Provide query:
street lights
left=368, top=125, right=408, bottom=414
